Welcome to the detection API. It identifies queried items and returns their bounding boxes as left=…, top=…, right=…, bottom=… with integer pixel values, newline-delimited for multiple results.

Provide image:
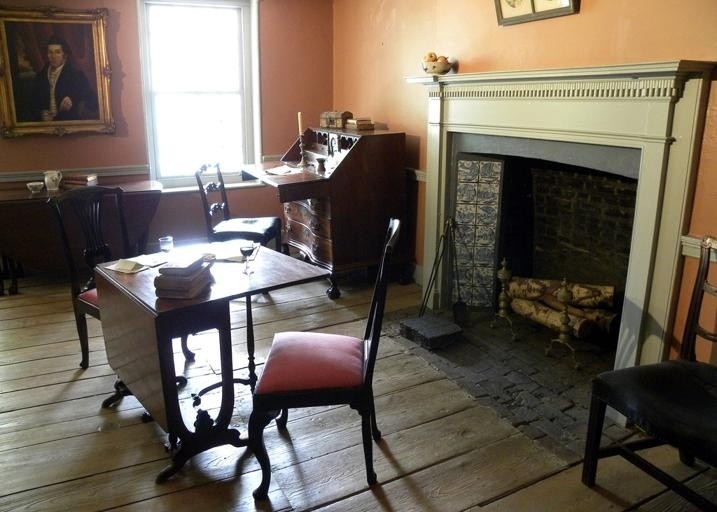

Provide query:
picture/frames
left=495, top=0, right=576, bottom=26
left=1, top=5, right=115, bottom=138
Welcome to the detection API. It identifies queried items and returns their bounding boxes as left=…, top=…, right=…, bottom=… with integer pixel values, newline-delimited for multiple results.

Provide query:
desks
left=92, top=237, right=334, bottom=484
left=1, top=180, right=163, bottom=296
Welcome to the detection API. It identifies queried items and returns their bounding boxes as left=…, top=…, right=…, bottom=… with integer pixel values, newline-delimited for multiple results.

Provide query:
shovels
left=449, top=217, right=468, bottom=321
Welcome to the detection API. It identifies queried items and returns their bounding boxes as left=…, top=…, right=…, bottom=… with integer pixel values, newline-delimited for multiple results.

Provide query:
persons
left=28, top=33, right=91, bottom=122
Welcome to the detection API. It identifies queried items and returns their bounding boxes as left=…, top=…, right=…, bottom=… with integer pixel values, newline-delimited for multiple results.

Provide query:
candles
left=298, top=111, right=303, bottom=136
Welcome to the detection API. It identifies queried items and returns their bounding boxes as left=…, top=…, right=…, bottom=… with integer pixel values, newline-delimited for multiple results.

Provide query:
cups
left=159, top=236, right=173, bottom=254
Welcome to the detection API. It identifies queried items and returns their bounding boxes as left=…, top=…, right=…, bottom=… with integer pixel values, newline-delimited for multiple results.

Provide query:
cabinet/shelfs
left=241, top=129, right=411, bottom=300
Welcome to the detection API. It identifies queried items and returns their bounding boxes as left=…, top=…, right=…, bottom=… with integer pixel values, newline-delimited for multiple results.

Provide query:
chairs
left=580, top=235, right=717, bottom=511
left=195, top=162, right=282, bottom=295
left=44, top=185, right=131, bottom=369
left=246, top=217, right=401, bottom=502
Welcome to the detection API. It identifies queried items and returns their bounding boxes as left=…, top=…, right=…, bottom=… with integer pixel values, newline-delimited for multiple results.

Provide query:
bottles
left=43, top=170, right=62, bottom=192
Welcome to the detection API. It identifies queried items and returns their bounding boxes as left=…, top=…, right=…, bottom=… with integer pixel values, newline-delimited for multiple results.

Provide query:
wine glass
left=238, top=238, right=255, bottom=275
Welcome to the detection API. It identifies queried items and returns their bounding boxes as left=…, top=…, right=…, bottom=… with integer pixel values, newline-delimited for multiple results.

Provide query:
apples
left=438, top=56, right=449, bottom=62
left=425, top=52, right=436, bottom=61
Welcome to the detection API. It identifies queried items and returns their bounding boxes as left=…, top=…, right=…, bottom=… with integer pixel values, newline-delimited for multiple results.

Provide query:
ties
left=48, top=69, right=58, bottom=117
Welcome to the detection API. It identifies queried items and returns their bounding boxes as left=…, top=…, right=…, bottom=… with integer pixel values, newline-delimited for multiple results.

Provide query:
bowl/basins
left=26, top=181, right=45, bottom=194
left=421, top=61, right=452, bottom=74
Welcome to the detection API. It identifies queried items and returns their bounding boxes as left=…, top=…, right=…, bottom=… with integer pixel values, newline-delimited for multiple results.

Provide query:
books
left=154, top=254, right=213, bottom=300
left=344, top=123, right=374, bottom=131
left=346, top=118, right=371, bottom=125
left=61, top=173, right=99, bottom=190
